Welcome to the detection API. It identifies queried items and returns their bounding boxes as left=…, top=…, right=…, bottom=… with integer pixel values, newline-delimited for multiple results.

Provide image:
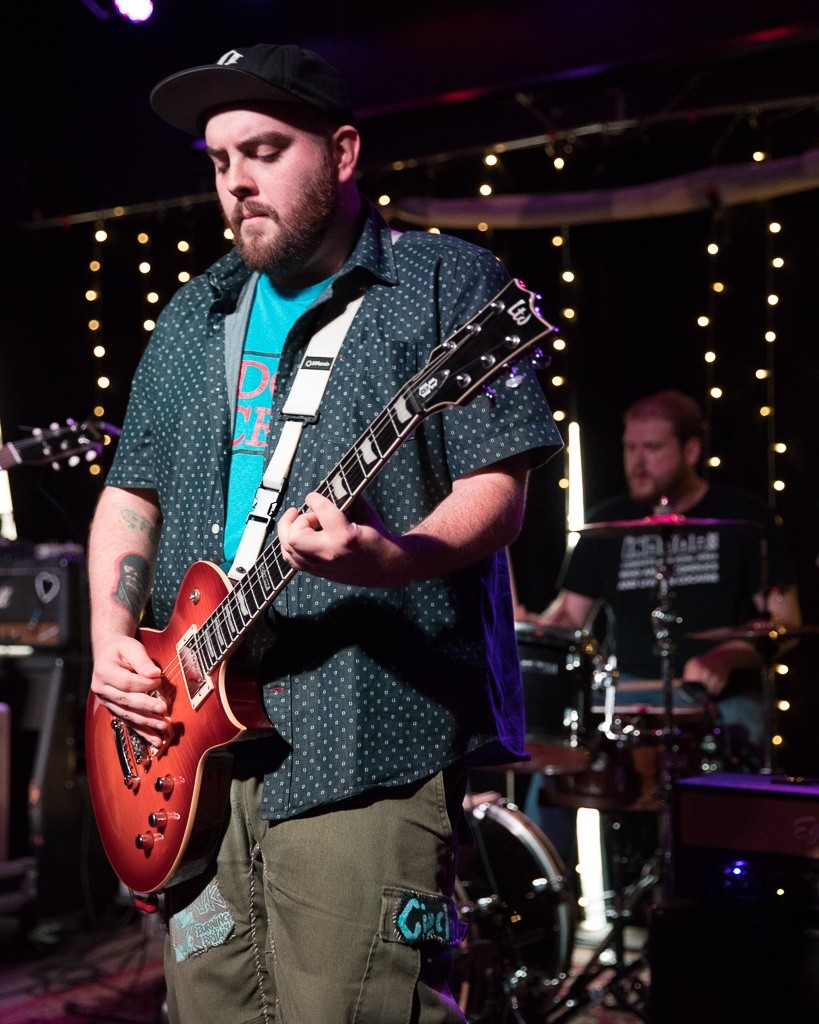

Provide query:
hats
left=149, top=43, right=355, bottom=136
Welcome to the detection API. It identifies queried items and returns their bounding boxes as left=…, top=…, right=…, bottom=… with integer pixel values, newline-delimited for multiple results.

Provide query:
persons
left=87, top=43, right=565, bottom=1023
left=514, top=393, right=803, bottom=1010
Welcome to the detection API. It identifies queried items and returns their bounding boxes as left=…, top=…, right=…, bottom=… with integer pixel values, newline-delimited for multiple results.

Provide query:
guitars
left=84, top=276, right=559, bottom=901
left=0, top=414, right=106, bottom=470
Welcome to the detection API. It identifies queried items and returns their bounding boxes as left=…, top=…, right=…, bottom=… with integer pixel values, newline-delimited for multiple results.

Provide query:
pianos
left=1, top=544, right=85, bottom=674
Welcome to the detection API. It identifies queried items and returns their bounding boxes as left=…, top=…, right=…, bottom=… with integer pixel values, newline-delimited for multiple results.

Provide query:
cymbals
left=563, top=513, right=748, bottom=534
left=673, top=617, right=819, bottom=654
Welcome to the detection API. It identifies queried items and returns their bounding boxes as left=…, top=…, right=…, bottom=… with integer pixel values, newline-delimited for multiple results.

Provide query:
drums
left=442, top=792, right=576, bottom=1000
left=462, top=616, right=591, bottom=778
left=533, top=702, right=726, bottom=812
